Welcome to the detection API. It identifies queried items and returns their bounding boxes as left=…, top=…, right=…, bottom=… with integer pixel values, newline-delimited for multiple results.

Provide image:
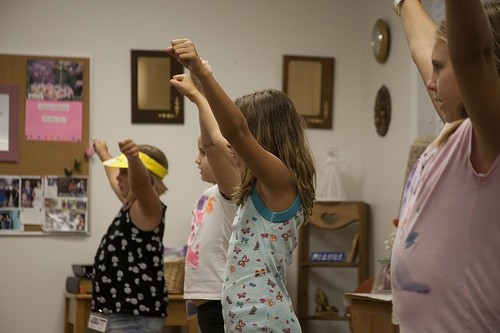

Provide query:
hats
left=102, top=149, right=168, bottom=179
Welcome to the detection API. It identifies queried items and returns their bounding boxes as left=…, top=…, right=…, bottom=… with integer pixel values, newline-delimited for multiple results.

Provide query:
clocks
left=371, top=19, right=389, bottom=63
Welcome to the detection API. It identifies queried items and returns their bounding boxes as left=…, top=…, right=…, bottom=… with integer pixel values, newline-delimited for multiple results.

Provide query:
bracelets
left=393, top=0, right=422, bottom=17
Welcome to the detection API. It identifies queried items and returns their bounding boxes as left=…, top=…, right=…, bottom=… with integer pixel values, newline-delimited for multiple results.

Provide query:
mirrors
left=282, top=55, right=335, bottom=130
left=130, top=49, right=184, bottom=125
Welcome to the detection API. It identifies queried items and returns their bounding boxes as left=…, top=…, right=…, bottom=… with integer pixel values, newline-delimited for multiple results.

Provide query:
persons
left=169, top=38, right=316, bottom=333
left=0, top=178, right=85, bottom=231
left=390, top=0, right=500, bottom=333
left=182, top=59, right=238, bottom=333
left=89, top=136, right=168, bottom=333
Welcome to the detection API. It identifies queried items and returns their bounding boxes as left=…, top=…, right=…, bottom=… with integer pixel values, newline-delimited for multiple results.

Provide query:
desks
left=343, top=293, right=400, bottom=333
left=63, top=292, right=208, bottom=333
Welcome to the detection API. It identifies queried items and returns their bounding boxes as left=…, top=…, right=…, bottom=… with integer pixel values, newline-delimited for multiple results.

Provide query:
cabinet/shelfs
left=295, top=200, right=374, bottom=333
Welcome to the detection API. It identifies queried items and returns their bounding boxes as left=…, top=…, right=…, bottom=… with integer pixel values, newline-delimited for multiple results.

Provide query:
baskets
left=162, top=256, right=185, bottom=295
